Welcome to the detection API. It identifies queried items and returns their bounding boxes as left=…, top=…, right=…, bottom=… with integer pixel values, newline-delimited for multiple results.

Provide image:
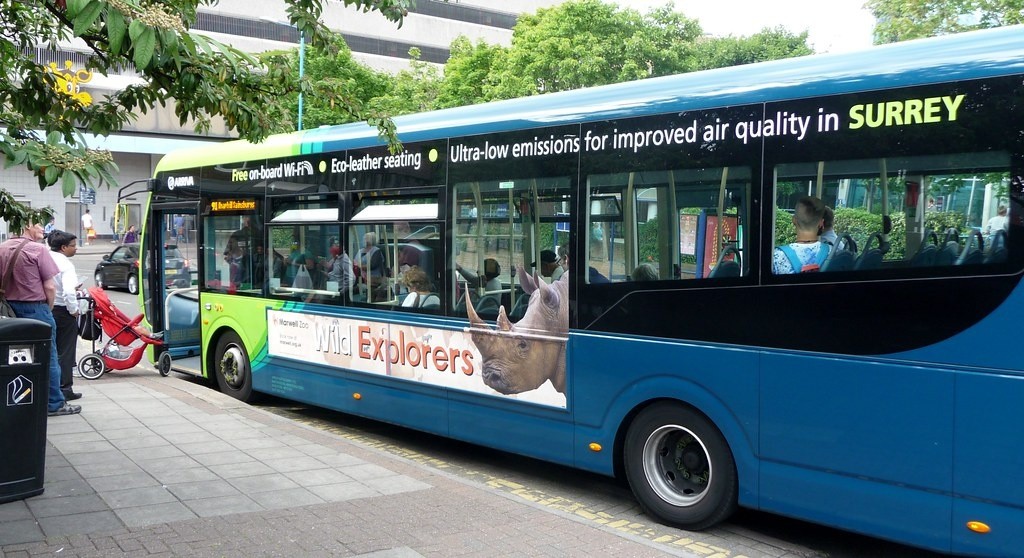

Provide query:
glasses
left=556, top=257, right=563, bottom=266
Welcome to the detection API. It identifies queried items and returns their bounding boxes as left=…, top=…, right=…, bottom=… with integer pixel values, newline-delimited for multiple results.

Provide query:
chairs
left=708, top=246, right=741, bottom=280
left=291, top=255, right=530, bottom=317
left=816, top=231, right=1007, bottom=274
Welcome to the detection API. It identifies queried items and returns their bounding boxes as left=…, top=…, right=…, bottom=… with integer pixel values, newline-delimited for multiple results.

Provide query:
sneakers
left=153, top=331, right=164, bottom=340
left=62, top=388, right=82, bottom=401
left=47, top=402, right=81, bottom=415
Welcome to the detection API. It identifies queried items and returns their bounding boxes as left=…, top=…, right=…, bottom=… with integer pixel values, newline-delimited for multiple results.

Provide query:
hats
left=530, top=250, right=557, bottom=267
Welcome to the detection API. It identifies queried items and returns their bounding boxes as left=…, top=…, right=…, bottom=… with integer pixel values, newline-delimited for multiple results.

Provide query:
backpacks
left=779, top=242, right=829, bottom=274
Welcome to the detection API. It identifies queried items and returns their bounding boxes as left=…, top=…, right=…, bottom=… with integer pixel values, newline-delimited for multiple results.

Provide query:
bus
left=112, top=22, right=1024, bottom=555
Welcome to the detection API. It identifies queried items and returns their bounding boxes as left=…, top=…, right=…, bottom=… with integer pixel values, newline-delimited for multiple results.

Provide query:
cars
left=93, top=242, right=191, bottom=295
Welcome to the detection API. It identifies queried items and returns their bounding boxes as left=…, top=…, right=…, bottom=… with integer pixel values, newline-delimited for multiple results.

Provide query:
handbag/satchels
left=0, top=296, right=17, bottom=318
left=88, top=229, right=97, bottom=239
left=78, top=300, right=102, bottom=344
left=292, top=265, right=314, bottom=297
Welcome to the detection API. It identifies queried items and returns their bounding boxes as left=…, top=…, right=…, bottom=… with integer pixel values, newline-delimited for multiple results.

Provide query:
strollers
left=77, top=286, right=164, bottom=381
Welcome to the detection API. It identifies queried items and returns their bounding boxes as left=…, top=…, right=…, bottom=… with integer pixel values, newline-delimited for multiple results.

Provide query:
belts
left=8, top=299, right=48, bottom=305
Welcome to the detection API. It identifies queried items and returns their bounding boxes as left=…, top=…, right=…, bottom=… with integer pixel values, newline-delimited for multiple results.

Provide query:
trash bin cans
left=0, top=318, right=53, bottom=504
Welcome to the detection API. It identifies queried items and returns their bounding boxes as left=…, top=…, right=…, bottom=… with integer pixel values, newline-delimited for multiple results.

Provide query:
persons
left=224, top=214, right=441, bottom=309
left=81, top=209, right=94, bottom=245
left=632, top=264, right=659, bottom=280
left=771, top=196, right=838, bottom=275
left=109, top=211, right=118, bottom=243
left=51, top=231, right=82, bottom=401
left=455, top=258, right=501, bottom=314
left=46, top=229, right=81, bottom=378
left=928, top=198, right=937, bottom=211
left=44, top=217, right=57, bottom=245
left=123, top=225, right=136, bottom=243
left=0, top=210, right=81, bottom=416
left=816, top=204, right=845, bottom=251
left=984, top=204, right=1009, bottom=249
left=509, top=250, right=564, bottom=317
left=555, top=243, right=611, bottom=282
left=175, top=213, right=184, bottom=247
